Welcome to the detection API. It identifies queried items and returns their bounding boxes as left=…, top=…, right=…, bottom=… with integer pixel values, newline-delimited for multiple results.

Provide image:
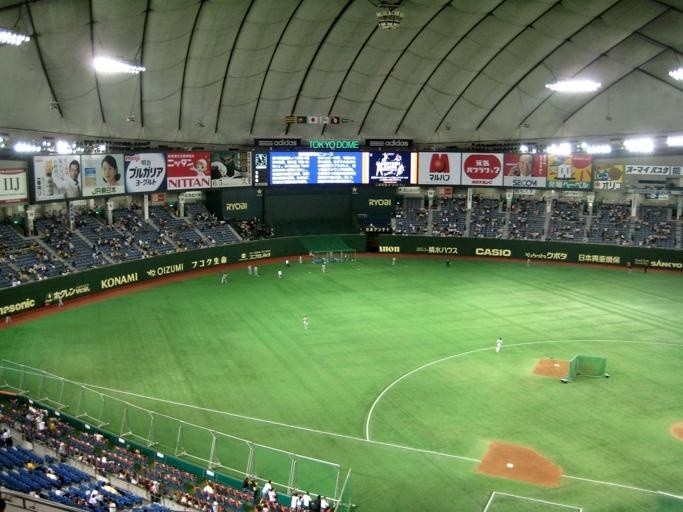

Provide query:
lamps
left=372, top=0, right=404, bottom=33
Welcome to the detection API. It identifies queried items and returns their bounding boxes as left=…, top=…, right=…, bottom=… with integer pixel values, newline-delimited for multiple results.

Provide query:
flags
left=308, top=115, right=317, bottom=124
left=330, top=115, right=340, bottom=124
left=297, top=115, right=307, bottom=124
left=285, top=115, right=295, bottom=123
left=319, top=115, right=329, bottom=124
left=342, top=117, right=349, bottom=123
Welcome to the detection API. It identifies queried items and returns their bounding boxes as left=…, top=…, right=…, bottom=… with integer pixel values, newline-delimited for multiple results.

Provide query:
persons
left=299, top=254, right=303, bottom=263
left=507, top=154, right=537, bottom=177
left=390, top=256, right=396, bottom=265
left=526, top=257, right=530, bottom=265
left=286, top=259, right=290, bottom=269
left=495, top=337, right=502, bottom=353
left=52, top=158, right=83, bottom=198
left=321, top=264, right=326, bottom=272
left=445, top=258, right=450, bottom=267
left=627, top=261, right=632, bottom=273
left=278, top=269, right=282, bottom=279
left=99, top=154, right=122, bottom=185
left=643, top=262, right=649, bottom=272
left=303, top=315, right=309, bottom=329
left=308, top=249, right=313, bottom=257
left=392, top=191, right=683, bottom=254
left=0, top=202, right=276, bottom=290
left=1, top=397, right=336, bottom=511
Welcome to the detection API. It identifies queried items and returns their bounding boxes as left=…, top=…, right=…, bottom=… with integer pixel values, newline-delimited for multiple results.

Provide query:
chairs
left=389, top=195, right=683, bottom=249
left=0, top=202, right=244, bottom=288
